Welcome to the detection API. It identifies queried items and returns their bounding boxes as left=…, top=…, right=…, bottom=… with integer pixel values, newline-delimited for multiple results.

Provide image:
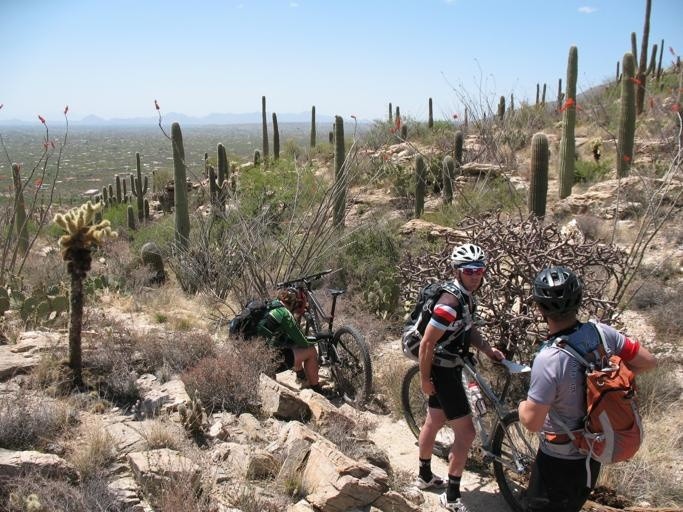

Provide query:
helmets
left=534, top=268, right=582, bottom=314
left=450, top=244, right=485, bottom=270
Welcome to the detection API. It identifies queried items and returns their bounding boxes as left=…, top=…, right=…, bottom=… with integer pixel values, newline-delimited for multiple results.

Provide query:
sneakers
left=416, top=475, right=449, bottom=490
left=297, top=371, right=306, bottom=378
left=312, top=383, right=338, bottom=400
left=438, top=492, right=472, bottom=512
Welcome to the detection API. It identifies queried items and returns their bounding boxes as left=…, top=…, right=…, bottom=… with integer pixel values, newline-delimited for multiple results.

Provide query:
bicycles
left=401, top=323, right=603, bottom=512
left=275, top=268, right=371, bottom=405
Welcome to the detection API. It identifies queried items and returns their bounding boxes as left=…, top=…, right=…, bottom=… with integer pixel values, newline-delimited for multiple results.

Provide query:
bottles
left=468, top=382, right=488, bottom=417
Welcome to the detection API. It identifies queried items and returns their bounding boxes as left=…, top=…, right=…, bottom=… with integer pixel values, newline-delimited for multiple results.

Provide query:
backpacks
left=548, top=319, right=642, bottom=464
left=229, top=300, right=283, bottom=337
left=402, top=282, right=476, bottom=360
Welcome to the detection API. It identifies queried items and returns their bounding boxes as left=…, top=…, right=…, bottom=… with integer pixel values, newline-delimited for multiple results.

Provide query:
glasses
left=459, top=268, right=482, bottom=275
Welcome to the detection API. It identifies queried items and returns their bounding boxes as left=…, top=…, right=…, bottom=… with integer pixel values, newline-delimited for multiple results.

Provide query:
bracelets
left=517, top=398, right=525, bottom=408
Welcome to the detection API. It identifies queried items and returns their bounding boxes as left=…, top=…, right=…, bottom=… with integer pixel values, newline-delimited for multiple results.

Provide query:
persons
left=517, top=266, right=658, bottom=512
left=255, top=287, right=338, bottom=399
left=415, top=243, right=505, bottom=512
